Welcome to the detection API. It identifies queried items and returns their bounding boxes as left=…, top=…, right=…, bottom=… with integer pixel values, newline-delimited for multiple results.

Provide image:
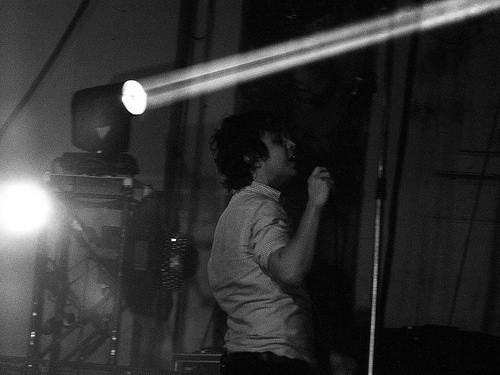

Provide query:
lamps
left=60, top=79, right=147, bottom=176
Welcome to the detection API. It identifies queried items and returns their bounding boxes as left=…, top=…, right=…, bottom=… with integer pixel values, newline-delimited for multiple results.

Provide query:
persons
left=208, top=111, right=332, bottom=375
